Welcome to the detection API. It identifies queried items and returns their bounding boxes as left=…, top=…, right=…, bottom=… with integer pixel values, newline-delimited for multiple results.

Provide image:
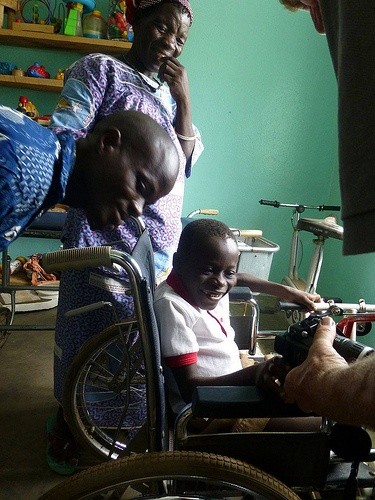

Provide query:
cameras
left=274, top=314, right=374, bottom=366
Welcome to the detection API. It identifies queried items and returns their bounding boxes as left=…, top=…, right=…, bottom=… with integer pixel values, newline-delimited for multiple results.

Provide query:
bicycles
left=258, top=198, right=344, bottom=328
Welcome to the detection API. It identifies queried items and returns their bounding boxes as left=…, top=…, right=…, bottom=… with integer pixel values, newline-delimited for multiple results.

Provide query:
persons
left=47, top=0, right=204, bottom=472
left=0, top=104, right=180, bottom=254
left=151, top=219, right=328, bottom=457
left=284, top=317, right=375, bottom=429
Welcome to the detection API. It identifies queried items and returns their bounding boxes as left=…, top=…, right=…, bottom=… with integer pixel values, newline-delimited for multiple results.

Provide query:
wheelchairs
left=41, top=244, right=374, bottom=500
left=180, top=207, right=286, bottom=362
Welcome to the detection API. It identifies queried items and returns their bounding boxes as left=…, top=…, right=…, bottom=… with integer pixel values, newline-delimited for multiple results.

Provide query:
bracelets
left=174, top=127, right=196, bottom=141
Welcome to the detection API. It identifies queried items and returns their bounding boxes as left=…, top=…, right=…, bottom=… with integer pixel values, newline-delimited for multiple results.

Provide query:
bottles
left=84, top=9, right=107, bottom=38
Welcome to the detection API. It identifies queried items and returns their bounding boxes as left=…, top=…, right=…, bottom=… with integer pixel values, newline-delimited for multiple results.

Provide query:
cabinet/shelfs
left=0, top=27, right=133, bottom=126
